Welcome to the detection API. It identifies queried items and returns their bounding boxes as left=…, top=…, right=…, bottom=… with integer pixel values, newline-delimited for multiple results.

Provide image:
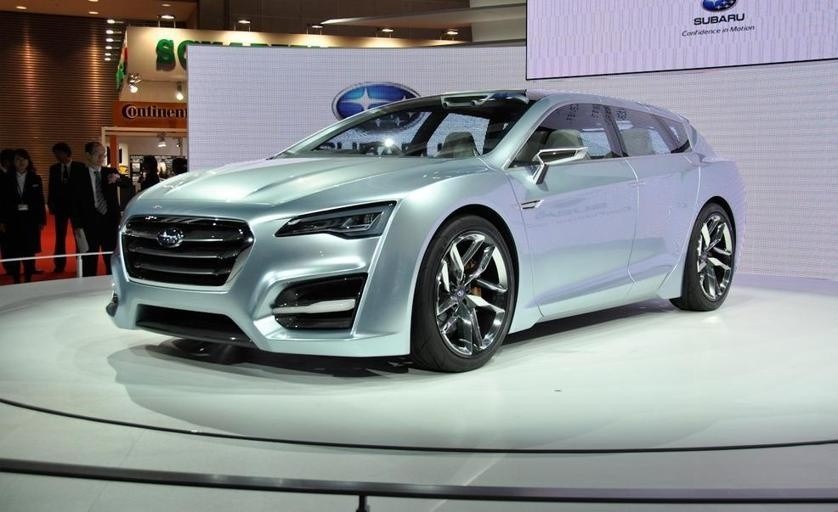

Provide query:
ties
left=63, top=164, right=69, bottom=183
left=95, top=171, right=107, bottom=215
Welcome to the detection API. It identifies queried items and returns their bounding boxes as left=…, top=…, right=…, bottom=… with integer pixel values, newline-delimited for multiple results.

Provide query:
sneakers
left=5, top=264, right=65, bottom=276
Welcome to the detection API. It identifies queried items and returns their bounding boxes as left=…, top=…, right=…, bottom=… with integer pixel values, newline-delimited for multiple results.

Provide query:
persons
left=0, top=140, right=188, bottom=285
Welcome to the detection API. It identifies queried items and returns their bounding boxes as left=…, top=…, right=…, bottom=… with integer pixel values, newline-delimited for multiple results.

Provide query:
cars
left=128, top=148, right=185, bottom=180
left=103, top=85, right=741, bottom=371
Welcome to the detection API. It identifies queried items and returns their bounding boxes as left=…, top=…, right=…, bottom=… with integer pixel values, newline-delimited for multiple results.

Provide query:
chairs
left=540, top=129, right=591, bottom=161
left=605, top=128, right=655, bottom=158
left=433, top=132, right=479, bottom=158
